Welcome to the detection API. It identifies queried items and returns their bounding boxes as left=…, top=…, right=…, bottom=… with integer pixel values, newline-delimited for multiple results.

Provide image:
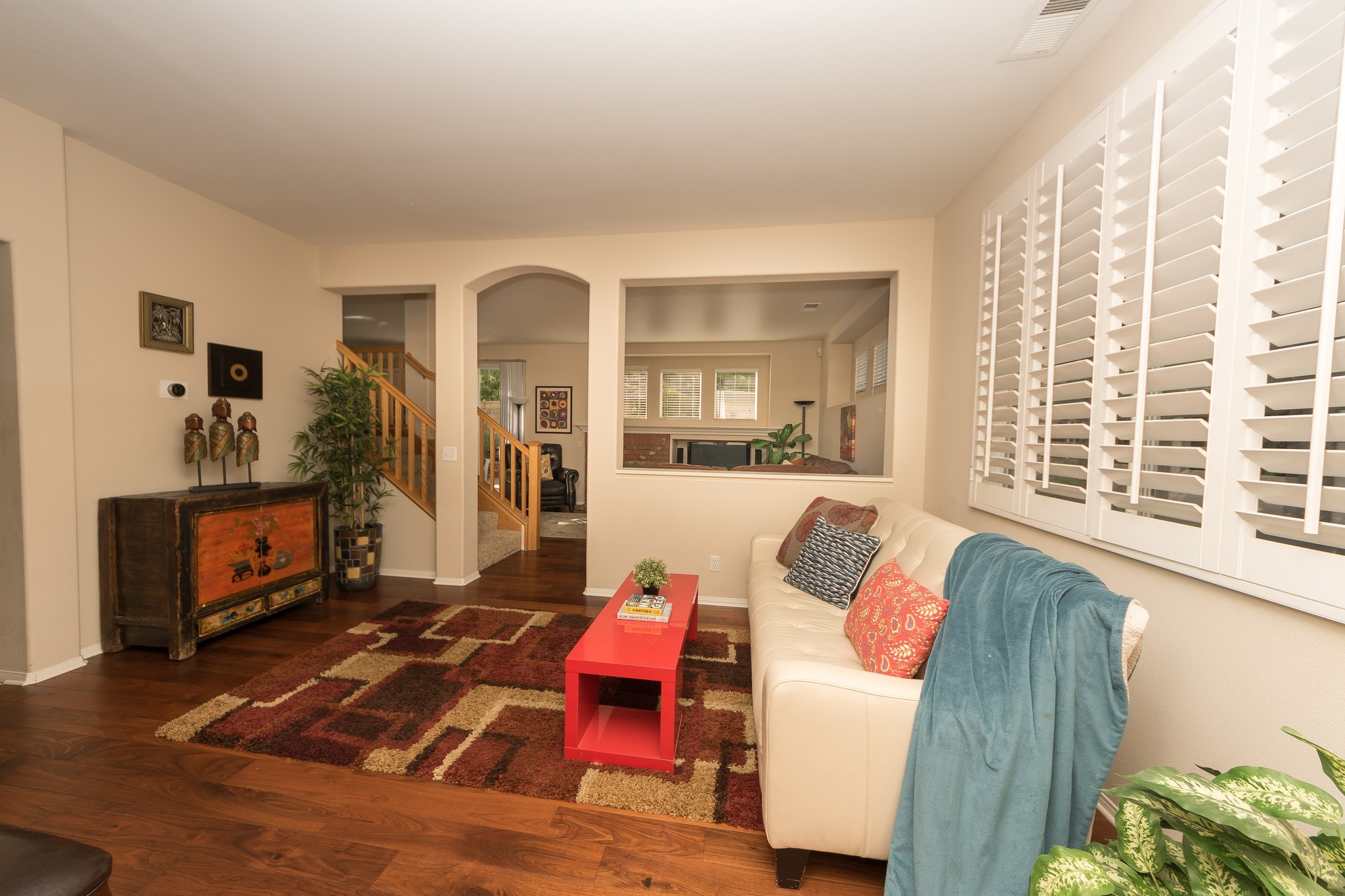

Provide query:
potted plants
left=630, top=554, right=672, bottom=595
left=286, top=353, right=400, bottom=593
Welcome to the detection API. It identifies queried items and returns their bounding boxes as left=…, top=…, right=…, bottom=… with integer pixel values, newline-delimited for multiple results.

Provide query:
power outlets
left=709, top=556, right=720, bottom=572
left=442, top=447, right=456, bottom=461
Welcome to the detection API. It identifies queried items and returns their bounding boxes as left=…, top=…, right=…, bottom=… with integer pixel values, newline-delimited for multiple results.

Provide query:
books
left=617, top=594, right=672, bottom=623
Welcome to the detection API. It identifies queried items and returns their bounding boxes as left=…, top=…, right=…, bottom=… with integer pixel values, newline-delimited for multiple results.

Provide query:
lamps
left=508, top=397, right=529, bottom=442
left=793, top=400, right=816, bottom=458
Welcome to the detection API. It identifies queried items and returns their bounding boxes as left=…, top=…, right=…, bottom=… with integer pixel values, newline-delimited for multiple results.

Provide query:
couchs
left=505, top=443, right=579, bottom=512
left=747, top=498, right=1147, bottom=889
left=623, top=455, right=858, bottom=474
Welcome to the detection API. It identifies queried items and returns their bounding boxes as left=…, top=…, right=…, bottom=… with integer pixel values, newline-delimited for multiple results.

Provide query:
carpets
left=540, top=511, right=587, bottom=539
left=155, top=600, right=764, bottom=833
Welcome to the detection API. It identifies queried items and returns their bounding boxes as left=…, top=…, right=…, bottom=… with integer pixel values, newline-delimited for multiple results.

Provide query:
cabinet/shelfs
left=99, top=481, right=329, bottom=661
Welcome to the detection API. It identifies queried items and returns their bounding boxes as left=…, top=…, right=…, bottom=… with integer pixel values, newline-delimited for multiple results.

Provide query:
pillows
left=525, top=454, right=554, bottom=481
left=776, top=496, right=950, bottom=679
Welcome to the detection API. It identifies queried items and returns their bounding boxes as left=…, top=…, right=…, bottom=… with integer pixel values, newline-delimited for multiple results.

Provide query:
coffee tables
left=565, top=571, right=699, bottom=773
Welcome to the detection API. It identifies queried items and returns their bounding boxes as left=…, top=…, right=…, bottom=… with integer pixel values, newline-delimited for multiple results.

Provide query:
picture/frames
left=139, top=290, right=194, bottom=354
left=535, top=386, right=572, bottom=434
